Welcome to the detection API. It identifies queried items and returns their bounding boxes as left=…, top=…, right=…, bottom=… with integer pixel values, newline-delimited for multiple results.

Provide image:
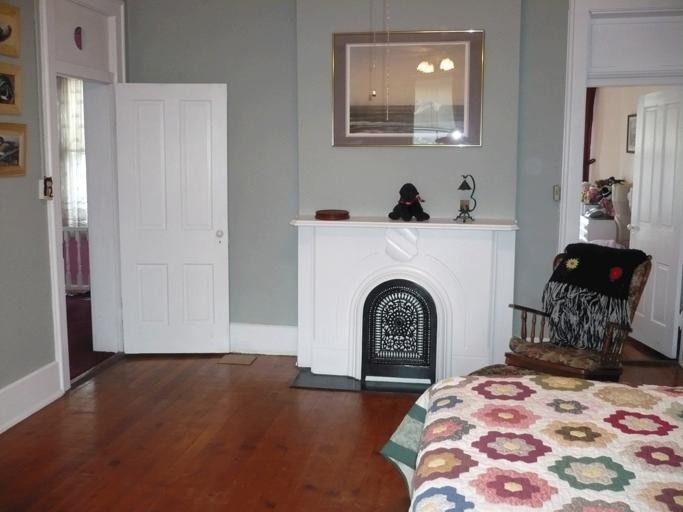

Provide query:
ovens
left=380, top=375, right=683, bottom=512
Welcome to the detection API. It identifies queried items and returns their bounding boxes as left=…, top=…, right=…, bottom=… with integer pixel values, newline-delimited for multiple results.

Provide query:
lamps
left=504, top=242, right=652, bottom=382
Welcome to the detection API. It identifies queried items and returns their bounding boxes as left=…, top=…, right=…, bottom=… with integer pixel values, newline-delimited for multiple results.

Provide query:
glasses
left=453, top=174, right=476, bottom=223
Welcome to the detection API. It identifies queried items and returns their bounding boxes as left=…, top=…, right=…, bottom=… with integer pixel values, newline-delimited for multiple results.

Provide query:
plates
left=580, top=215, right=617, bottom=243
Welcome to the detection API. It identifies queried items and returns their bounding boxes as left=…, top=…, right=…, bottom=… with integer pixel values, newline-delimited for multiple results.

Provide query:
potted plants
left=0, top=0, right=21, bottom=58
left=331, top=29, right=485, bottom=147
left=626, top=114, right=637, bottom=153
left=0, top=62, right=22, bottom=115
left=0, top=123, right=27, bottom=177
